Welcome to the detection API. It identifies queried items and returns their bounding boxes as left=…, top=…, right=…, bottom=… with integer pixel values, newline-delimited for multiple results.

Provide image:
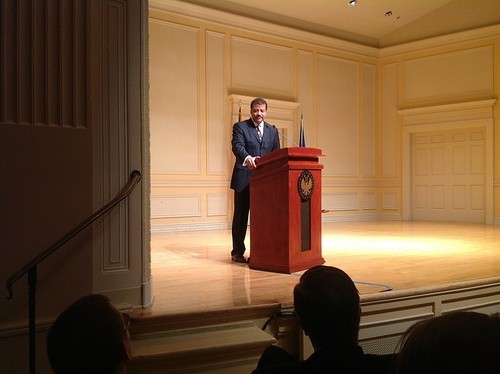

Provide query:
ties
left=256, top=125, right=263, bottom=143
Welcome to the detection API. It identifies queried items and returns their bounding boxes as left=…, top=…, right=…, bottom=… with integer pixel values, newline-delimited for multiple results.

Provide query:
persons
left=230, top=99, right=280, bottom=263
left=47, top=294, right=133, bottom=374
left=393, top=310, right=500, bottom=374
left=253, top=266, right=403, bottom=374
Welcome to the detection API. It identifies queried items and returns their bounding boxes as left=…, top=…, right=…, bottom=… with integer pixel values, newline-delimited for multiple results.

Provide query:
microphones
left=273, top=125, right=298, bottom=146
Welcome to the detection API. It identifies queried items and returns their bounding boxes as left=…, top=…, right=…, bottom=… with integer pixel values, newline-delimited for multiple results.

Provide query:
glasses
left=121, top=312, right=131, bottom=334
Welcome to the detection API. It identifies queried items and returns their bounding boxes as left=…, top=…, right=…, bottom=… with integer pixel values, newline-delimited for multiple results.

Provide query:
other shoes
left=232, top=254, right=247, bottom=263
